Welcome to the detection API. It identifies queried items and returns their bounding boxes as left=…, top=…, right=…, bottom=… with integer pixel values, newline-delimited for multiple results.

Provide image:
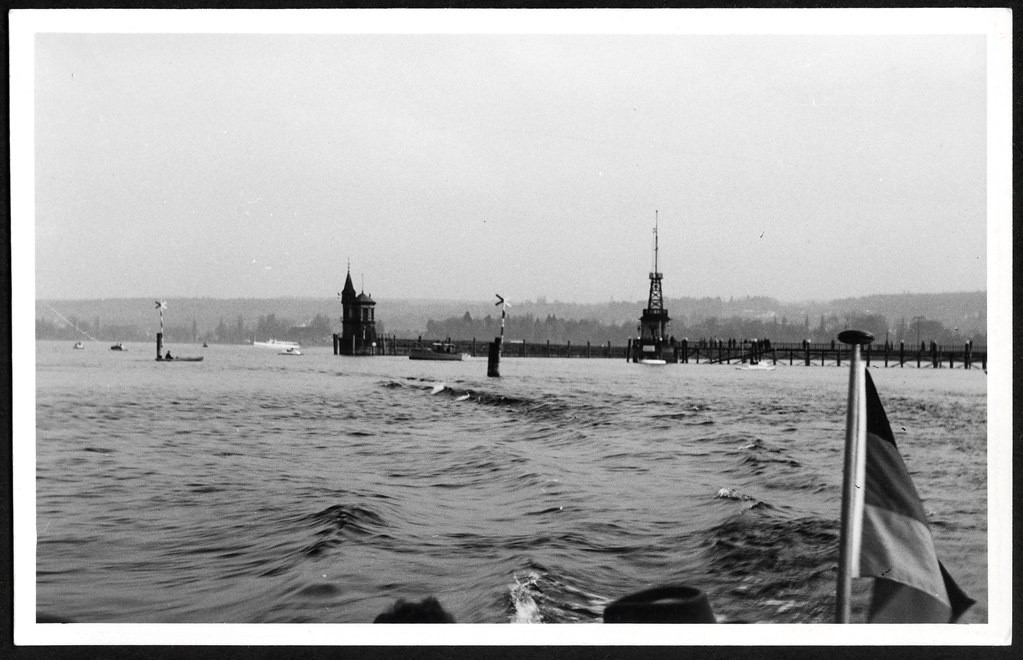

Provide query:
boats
left=156, top=354, right=205, bottom=363
left=110, top=345, right=126, bottom=352
left=277, top=348, right=302, bottom=356
left=409, top=337, right=463, bottom=361
left=74, top=342, right=85, bottom=350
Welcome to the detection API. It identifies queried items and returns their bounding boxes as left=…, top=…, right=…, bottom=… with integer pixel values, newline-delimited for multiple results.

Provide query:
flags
left=854, top=366, right=978, bottom=625
